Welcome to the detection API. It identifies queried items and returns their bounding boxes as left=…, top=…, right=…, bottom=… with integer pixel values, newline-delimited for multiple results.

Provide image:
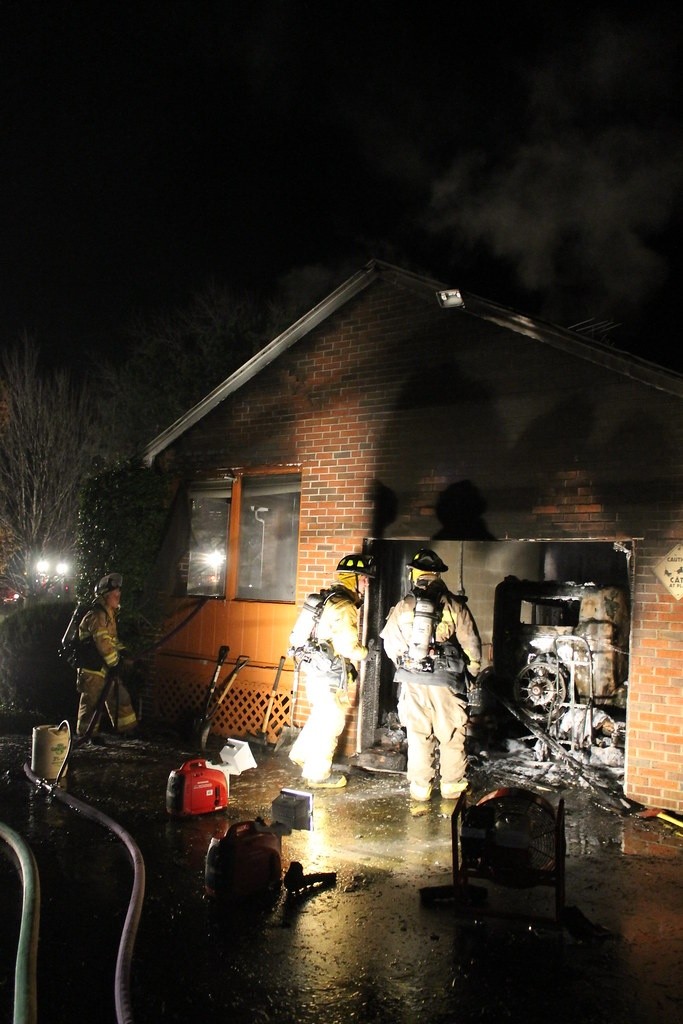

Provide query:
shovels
left=178, top=645, right=250, bottom=751
left=274, top=660, right=303, bottom=753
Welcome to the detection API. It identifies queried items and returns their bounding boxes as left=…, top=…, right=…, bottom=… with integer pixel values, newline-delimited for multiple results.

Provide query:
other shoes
left=289, top=747, right=306, bottom=768
left=305, top=774, right=347, bottom=789
left=410, top=787, right=432, bottom=801
left=440, top=787, right=462, bottom=798
left=124, top=729, right=148, bottom=740
left=78, top=736, right=104, bottom=745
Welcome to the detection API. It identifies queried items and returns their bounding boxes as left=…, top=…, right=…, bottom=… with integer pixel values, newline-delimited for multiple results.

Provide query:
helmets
left=93, top=572, right=123, bottom=598
left=336, top=555, right=377, bottom=578
left=406, top=548, right=448, bottom=572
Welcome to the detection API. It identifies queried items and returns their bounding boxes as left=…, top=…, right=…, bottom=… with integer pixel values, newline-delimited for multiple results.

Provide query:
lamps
left=163, top=737, right=258, bottom=814
left=203, top=786, right=314, bottom=899
left=434, top=288, right=468, bottom=310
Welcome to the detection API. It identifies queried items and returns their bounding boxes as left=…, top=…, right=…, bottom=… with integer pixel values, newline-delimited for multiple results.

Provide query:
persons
left=288, top=554, right=382, bottom=789
left=379, top=549, right=482, bottom=801
left=69, top=573, right=140, bottom=750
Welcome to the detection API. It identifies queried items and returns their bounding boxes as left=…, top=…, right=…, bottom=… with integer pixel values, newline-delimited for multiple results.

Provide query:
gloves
left=112, top=658, right=123, bottom=673
left=364, top=638, right=381, bottom=661
left=119, top=649, right=129, bottom=657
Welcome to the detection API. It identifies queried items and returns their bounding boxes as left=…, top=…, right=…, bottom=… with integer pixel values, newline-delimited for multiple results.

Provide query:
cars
left=490, top=577, right=627, bottom=725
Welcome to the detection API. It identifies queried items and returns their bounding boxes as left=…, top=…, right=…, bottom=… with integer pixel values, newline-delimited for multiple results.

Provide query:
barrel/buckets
left=31, top=724, right=71, bottom=779
